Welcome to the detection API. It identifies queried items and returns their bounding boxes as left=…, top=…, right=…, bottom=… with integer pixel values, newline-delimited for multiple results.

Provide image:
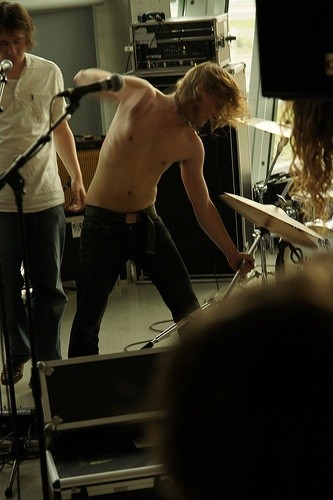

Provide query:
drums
left=275, top=216, right=333, bottom=288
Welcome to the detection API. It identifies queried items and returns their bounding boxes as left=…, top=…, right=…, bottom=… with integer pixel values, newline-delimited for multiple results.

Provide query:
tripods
left=0, top=273, right=40, bottom=497
left=141, top=183, right=276, bottom=350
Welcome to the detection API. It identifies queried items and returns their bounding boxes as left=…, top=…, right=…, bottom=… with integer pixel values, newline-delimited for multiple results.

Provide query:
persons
left=1, top=1, right=88, bottom=385
left=164, top=0, right=333, bottom=494
left=68, top=61, right=253, bottom=359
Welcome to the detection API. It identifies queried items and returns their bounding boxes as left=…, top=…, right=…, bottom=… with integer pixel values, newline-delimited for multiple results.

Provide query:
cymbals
left=235, top=115, right=294, bottom=139
left=219, top=189, right=325, bottom=250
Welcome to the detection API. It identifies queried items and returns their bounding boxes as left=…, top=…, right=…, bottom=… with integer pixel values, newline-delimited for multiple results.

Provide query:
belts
left=85, top=204, right=157, bottom=224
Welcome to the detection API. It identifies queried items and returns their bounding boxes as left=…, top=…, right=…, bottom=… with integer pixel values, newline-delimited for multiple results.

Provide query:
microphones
left=65, top=181, right=71, bottom=189
left=0, top=59, right=14, bottom=74
left=57, top=74, right=124, bottom=98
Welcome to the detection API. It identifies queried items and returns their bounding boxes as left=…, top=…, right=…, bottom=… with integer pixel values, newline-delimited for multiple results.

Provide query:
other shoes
left=1, top=358, right=30, bottom=385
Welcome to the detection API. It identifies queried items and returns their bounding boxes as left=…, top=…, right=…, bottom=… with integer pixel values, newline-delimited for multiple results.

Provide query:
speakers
left=255, top=0, right=333, bottom=99
left=56, top=139, right=105, bottom=216
left=130, top=106, right=248, bottom=283
